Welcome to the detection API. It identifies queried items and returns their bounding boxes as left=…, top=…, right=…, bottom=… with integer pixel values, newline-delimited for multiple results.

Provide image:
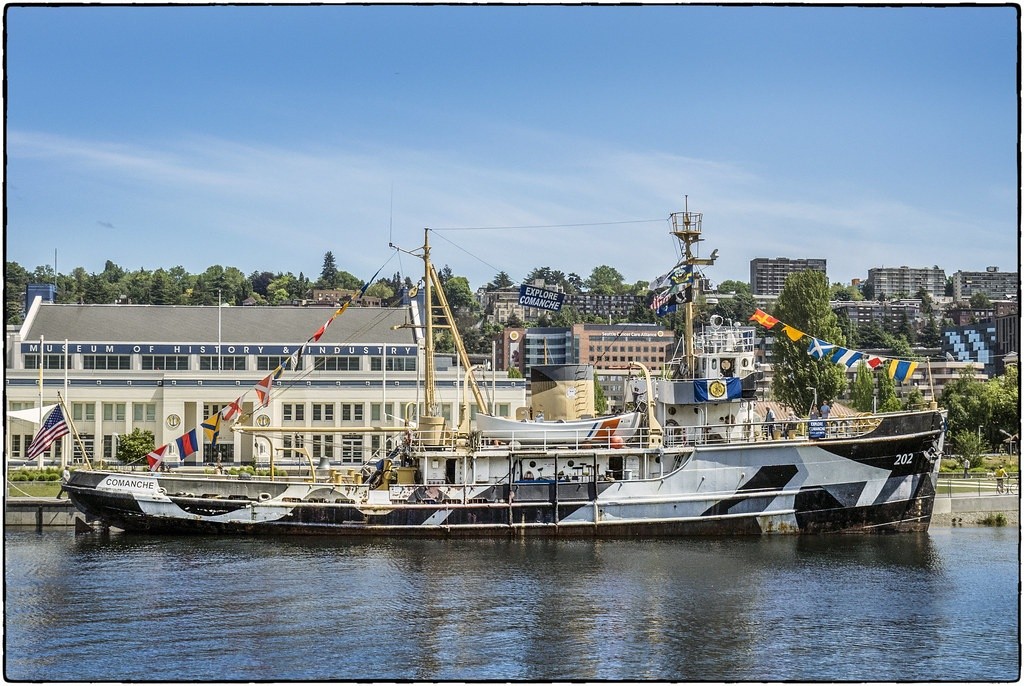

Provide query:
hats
left=823, top=400, right=828, bottom=404
left=999, top=465, right=1003, bottom=468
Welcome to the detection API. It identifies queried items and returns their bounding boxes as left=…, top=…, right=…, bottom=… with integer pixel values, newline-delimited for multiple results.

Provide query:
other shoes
left=969, top=476, right=973, bottom=479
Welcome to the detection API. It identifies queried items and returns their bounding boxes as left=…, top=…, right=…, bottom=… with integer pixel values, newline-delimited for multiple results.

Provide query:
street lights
left=978, top=425, right=984, bottom=457
left=805, top=387, right=817, bottom=408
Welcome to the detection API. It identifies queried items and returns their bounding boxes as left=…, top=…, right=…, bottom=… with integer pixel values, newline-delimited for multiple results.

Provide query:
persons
left=764, top=407, right=775, bottom=439
left=963, top=457, right=972, bottom=478
left=783, top=412, right=800, bottom=439
left=56, top=466, right=71, bottom=499
left=632, top=387, right=647, bottom=413
left=820, top=401, right=830, bottom=418
left=996, top=465, right=1008, bottom=494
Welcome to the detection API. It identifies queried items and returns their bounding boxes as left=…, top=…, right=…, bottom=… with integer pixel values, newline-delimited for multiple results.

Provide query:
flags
left=271, top=342, right=307, bottom=380
left=255, top=376, right=273, bottom=407
left=202, top=412, right=223, bottom=446
left=649, top=265, right=694, bottom=316
left=694, top=378, right=742, bottom=402
left=147, top=444, right=169, bottom=472
left=28, top=405, right=71, bottom=460
left=176, top=429, right=199, bottom=460
left=749, top=308, right=919, bottom=383
left=222, top=397, right=243, bottom=420
left=313, top=284, right=369, bottom=341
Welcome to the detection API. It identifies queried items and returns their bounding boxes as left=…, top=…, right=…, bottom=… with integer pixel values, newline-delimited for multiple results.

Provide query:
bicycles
left=997, top=477, right=1020, bottom=495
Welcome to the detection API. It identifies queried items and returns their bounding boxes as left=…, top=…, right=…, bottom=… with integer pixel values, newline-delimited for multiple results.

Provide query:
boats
left=475, top=411, right=642, bottom=446
left=61, top=196, right=950, bottom=537
